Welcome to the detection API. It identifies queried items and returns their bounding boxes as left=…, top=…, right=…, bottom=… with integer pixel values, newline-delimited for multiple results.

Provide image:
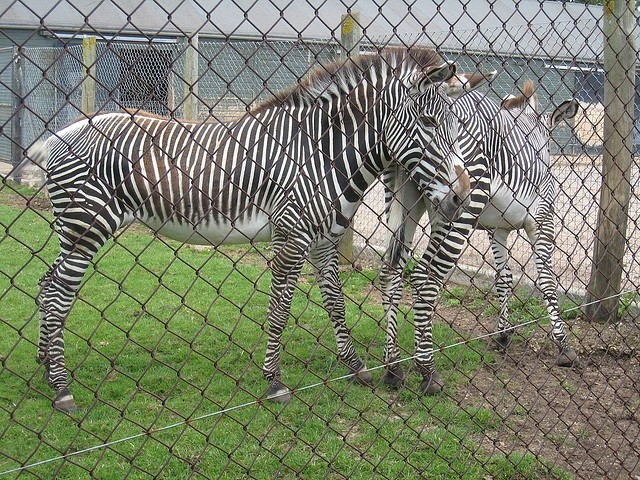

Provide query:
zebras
left=378, top=78, right=582, bottom=398
left=28, top=43, right=499, bottom=416
